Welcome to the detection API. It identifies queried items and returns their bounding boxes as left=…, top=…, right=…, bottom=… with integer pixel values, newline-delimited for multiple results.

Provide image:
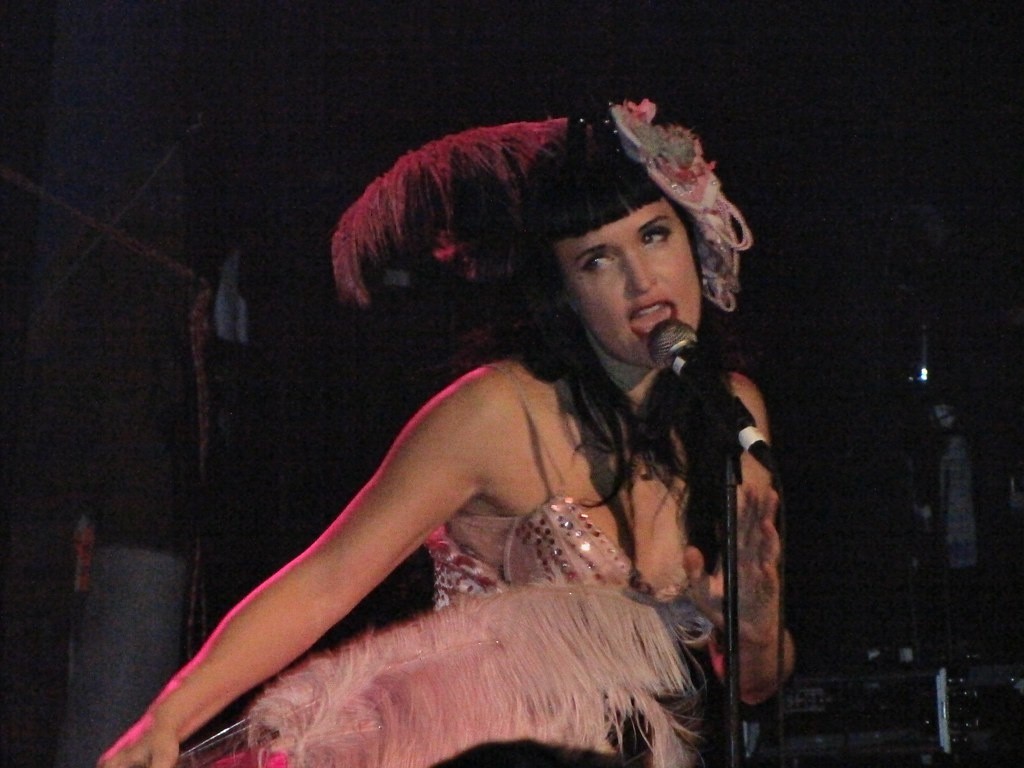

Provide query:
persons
left=95, top=100, right=794, bottom=768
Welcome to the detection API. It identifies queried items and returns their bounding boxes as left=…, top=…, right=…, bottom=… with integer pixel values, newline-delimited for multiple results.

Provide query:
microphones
left=646, top=318, right=785, bottom=470
